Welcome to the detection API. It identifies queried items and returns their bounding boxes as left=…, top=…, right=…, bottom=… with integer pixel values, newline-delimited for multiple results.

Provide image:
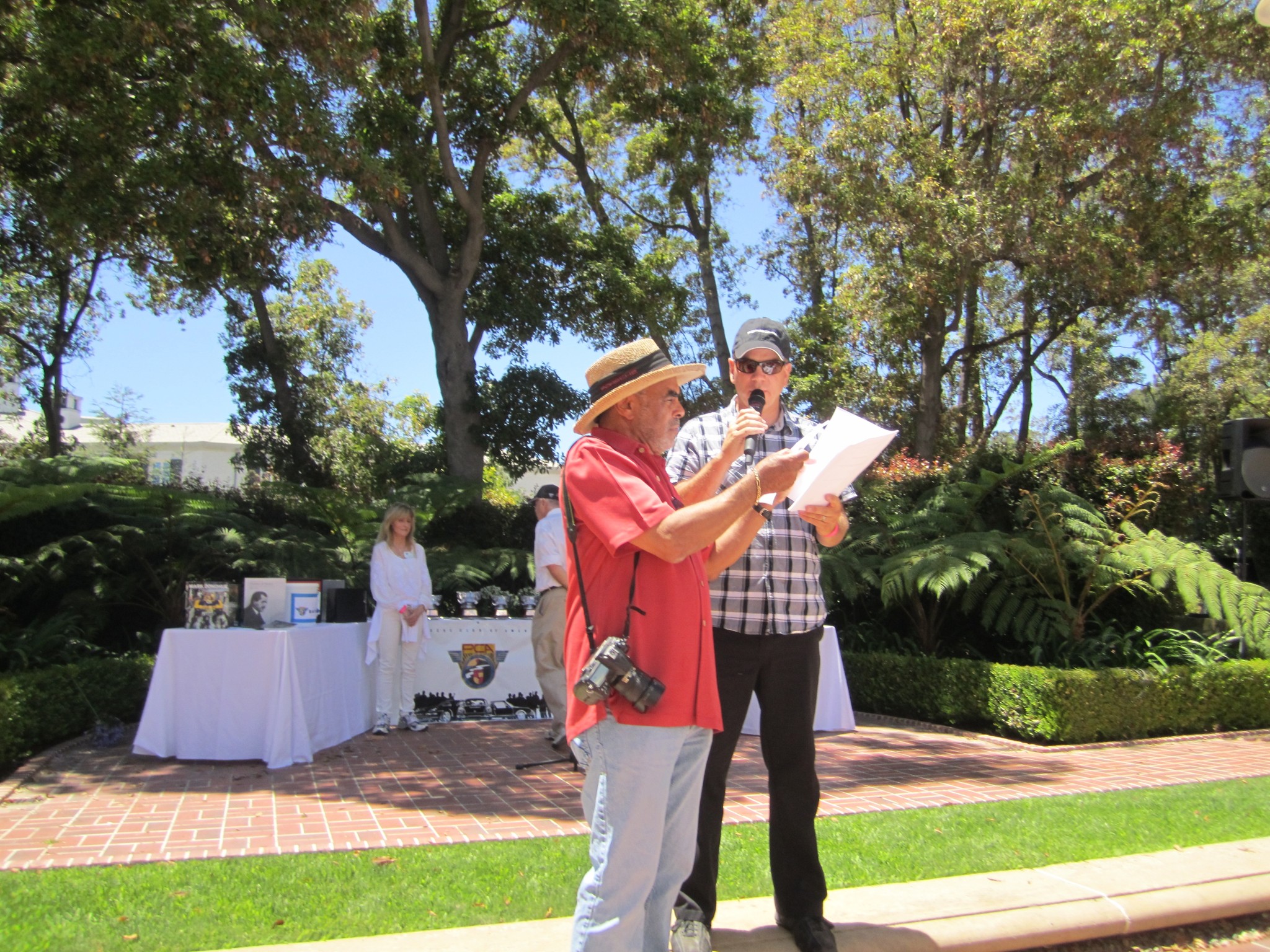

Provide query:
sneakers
left=776, top=917, right=837, bottom=952
left=398, top=718, right=428, bottom=731
left=372, top=714, right=391, bottom=734
left=670, top=918, right=712, bottom=952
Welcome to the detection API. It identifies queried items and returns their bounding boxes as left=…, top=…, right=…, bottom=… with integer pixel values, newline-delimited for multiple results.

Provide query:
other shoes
left=544, top=730, right=554, bottom=739
left=552, top=731, right=567, bottom=745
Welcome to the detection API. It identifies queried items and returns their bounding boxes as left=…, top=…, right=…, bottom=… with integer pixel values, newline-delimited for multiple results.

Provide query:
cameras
left=573, top=635, right=667, bottom=714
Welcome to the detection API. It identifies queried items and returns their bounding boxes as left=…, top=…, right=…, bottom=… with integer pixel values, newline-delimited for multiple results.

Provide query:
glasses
left=735, top=357, right=788, bottom=375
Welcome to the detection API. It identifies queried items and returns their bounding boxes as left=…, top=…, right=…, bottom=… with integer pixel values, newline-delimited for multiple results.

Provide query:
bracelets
left=752, top=468, right=761, bottom=505
left=399, top=606, right=406, bottom=613
left=752, top=504, right=772, bottom=522
left=823, top=523, right=839, bottom=537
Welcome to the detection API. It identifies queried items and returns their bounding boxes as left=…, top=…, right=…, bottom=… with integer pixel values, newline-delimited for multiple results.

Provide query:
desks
left=411, top=617, right=555, bottom=715
left=737, top=617, right=873, bottom=739
left=149, top=619, right=381, bottom=769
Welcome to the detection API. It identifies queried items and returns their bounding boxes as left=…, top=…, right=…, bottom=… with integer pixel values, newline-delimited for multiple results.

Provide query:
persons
left=365, top=502, right=432, bottom=735
left=243, top=591, right=268, bottom=626
left=666, top=317, right=857, bottom=952
left=528, top=484, right=567, bottom=749
left=559, top=339, right=808, bottom=952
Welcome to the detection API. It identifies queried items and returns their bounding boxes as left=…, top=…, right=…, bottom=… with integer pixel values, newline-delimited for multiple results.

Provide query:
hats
left=573, top=338, right=706, bottom=435
left=732, top=317, right=791, bottom=363
left=528, top=484, right=559, bottom=502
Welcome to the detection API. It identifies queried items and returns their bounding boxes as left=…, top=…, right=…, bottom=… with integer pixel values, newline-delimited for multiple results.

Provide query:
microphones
left=746, top=388, right=764, bottom=455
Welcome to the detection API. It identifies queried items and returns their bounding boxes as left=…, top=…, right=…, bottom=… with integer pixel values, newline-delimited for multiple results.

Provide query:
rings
left=822, top=516, right=826, bottom=522
